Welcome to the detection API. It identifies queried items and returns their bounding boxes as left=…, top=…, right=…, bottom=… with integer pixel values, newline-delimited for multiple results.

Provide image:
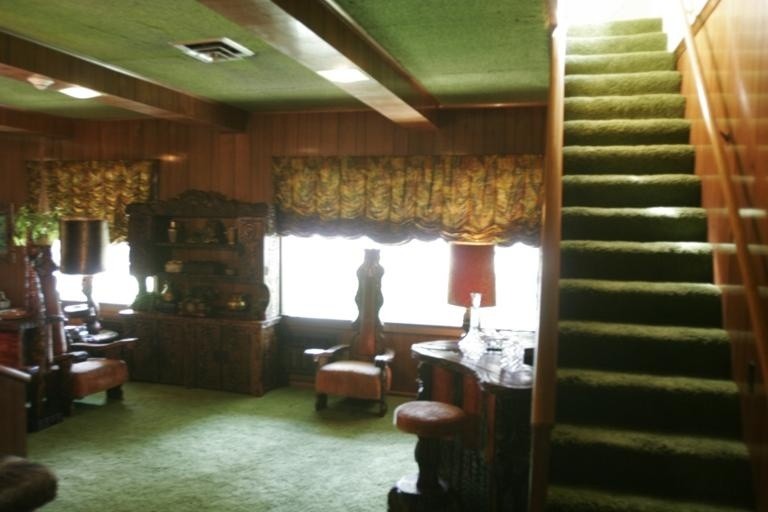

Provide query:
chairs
left=48, top=314, right=138, bottom=422
left=305, top=249, right=394, bottom=417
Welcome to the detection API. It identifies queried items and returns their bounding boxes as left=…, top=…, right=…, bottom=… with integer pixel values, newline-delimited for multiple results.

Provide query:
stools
left=387, top=400, right=468, bottom=512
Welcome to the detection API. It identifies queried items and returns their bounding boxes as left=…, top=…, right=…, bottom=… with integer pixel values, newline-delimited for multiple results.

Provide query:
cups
left=499, top=339, right=524, bottom=374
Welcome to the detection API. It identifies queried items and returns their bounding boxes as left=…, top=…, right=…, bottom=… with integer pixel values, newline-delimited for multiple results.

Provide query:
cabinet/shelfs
left=118, top=188, right=291, bottom=396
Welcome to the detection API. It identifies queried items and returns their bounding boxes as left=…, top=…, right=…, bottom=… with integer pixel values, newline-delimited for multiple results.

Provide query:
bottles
left=457, top=292, right=489, bottom=361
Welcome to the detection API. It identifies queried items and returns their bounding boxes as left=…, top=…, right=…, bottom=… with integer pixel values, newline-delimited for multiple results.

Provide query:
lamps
left=58, top=216, right=114, bottom=336
left=447, top=240, right=497, bottom=337
